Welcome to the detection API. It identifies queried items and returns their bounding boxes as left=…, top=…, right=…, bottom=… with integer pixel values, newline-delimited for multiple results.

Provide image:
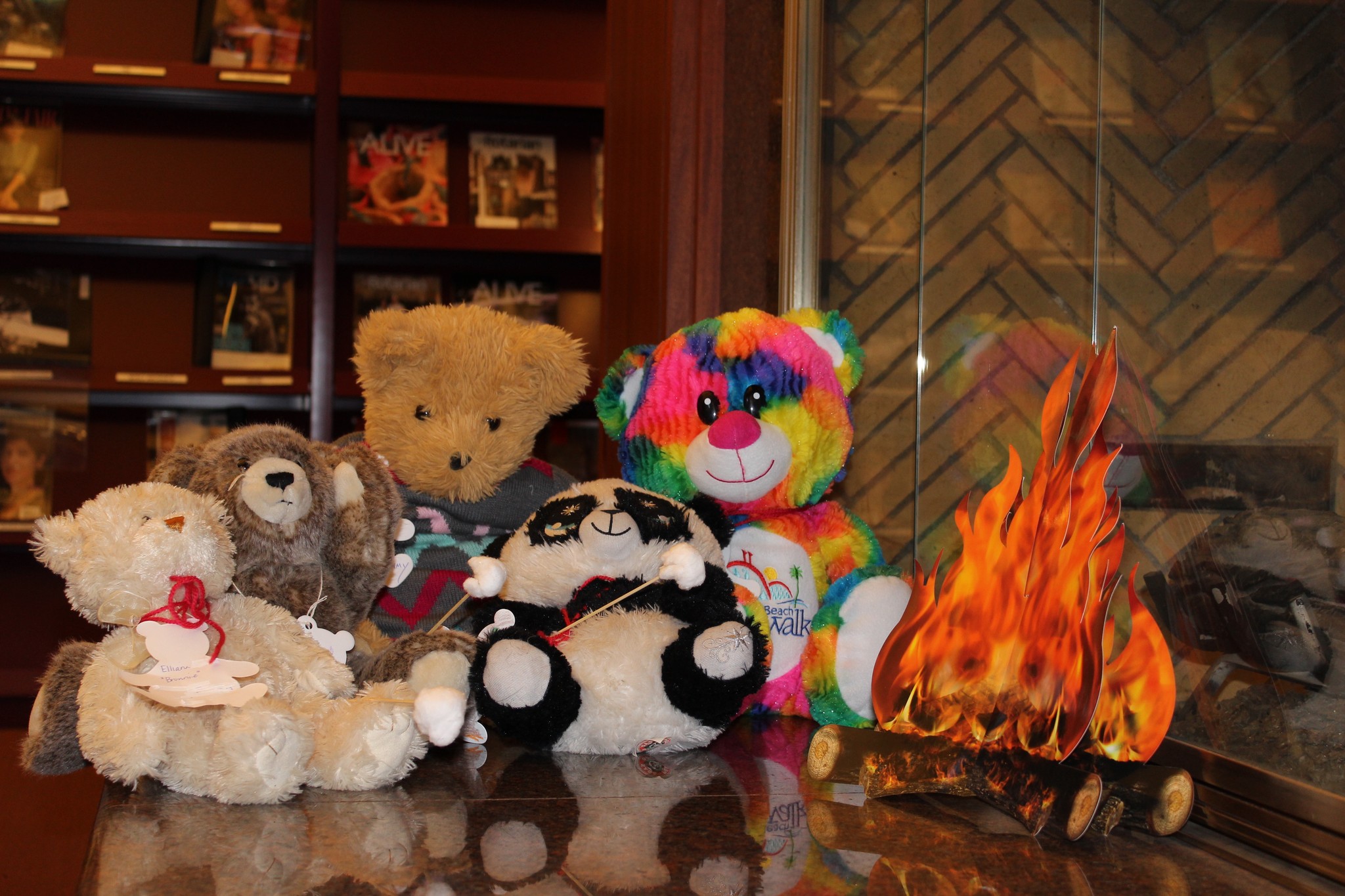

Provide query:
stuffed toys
left=590, top=306, right=914, bottom=731
left=15, top=424, right=402, bottom=779
left=26, top=480, right=427, bottom=807
left=77, top=716, right=854, bottom=896
left=326, top=302, right=591, bottom=752
left=465, top=476, right=773, bottom=758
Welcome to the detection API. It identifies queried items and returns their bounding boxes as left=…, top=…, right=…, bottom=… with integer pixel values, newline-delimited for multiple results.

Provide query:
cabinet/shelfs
left=0, top=0, right=608, bottom=531
